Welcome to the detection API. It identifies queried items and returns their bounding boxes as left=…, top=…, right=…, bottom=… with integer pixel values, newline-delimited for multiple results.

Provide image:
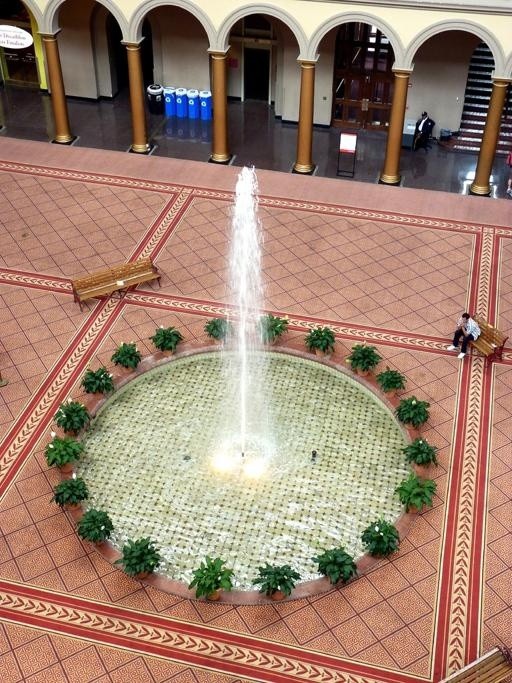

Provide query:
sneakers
left=447, top=344, right=459, bottom=351
left=458, top=352, right=466, bottom=358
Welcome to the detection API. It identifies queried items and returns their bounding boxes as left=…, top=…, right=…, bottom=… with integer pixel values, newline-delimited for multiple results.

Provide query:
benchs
left=469, top=314, right=508, bottom=364
left=428, top=645, right=509, bottom=681
left=71, top=257, right=161, bottom=314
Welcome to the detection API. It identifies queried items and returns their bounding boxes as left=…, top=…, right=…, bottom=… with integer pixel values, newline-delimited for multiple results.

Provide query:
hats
left=421, top=112, right=427, bottom=116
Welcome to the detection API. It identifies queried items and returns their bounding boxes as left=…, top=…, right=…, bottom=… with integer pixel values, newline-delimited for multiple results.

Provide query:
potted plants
left=188, top=554, right=236, bottom=602
left=74, top=508, right=115, bottom=546
left=309, top=546, right=360, bottom=585
left=50, top=478, right=91, bottom=511
left=147, top=325, right=184, bottom=357
left=344, top=341, right=382, bottom=377
left=394, top=473, right=437, bottom=513
left=376, top=366, right=406, bottom=398
left=43, top=433, right=86, bottom=474
left=302, top=326, right=336, bottom=358
left=111, top=341, right=138, bottom=373
left=113, top=536, right=162, bottom=579
left=392, top=396, right=431, bottom=430
left=51, top=399, right=92, bottom=437
left=250, top=561, right=301, bottom=602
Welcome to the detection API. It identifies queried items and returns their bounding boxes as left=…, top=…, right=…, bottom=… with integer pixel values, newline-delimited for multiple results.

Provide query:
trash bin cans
left=147, top=84, right=212, bottom=121
left=402, top=120, right=417, bottom=148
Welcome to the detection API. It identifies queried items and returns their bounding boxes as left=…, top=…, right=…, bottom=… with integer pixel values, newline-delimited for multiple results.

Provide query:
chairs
left=418, top=119, right=434, bottom=152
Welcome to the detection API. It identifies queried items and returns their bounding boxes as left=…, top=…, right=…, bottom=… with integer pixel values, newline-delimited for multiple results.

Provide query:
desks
left=403, top=119, right=415, bottom=151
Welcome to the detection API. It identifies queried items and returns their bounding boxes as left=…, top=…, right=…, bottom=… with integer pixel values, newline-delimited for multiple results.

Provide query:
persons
left=446, top=313, right=481, bottom=360
left=412, top=112, right=434, bottom=152
